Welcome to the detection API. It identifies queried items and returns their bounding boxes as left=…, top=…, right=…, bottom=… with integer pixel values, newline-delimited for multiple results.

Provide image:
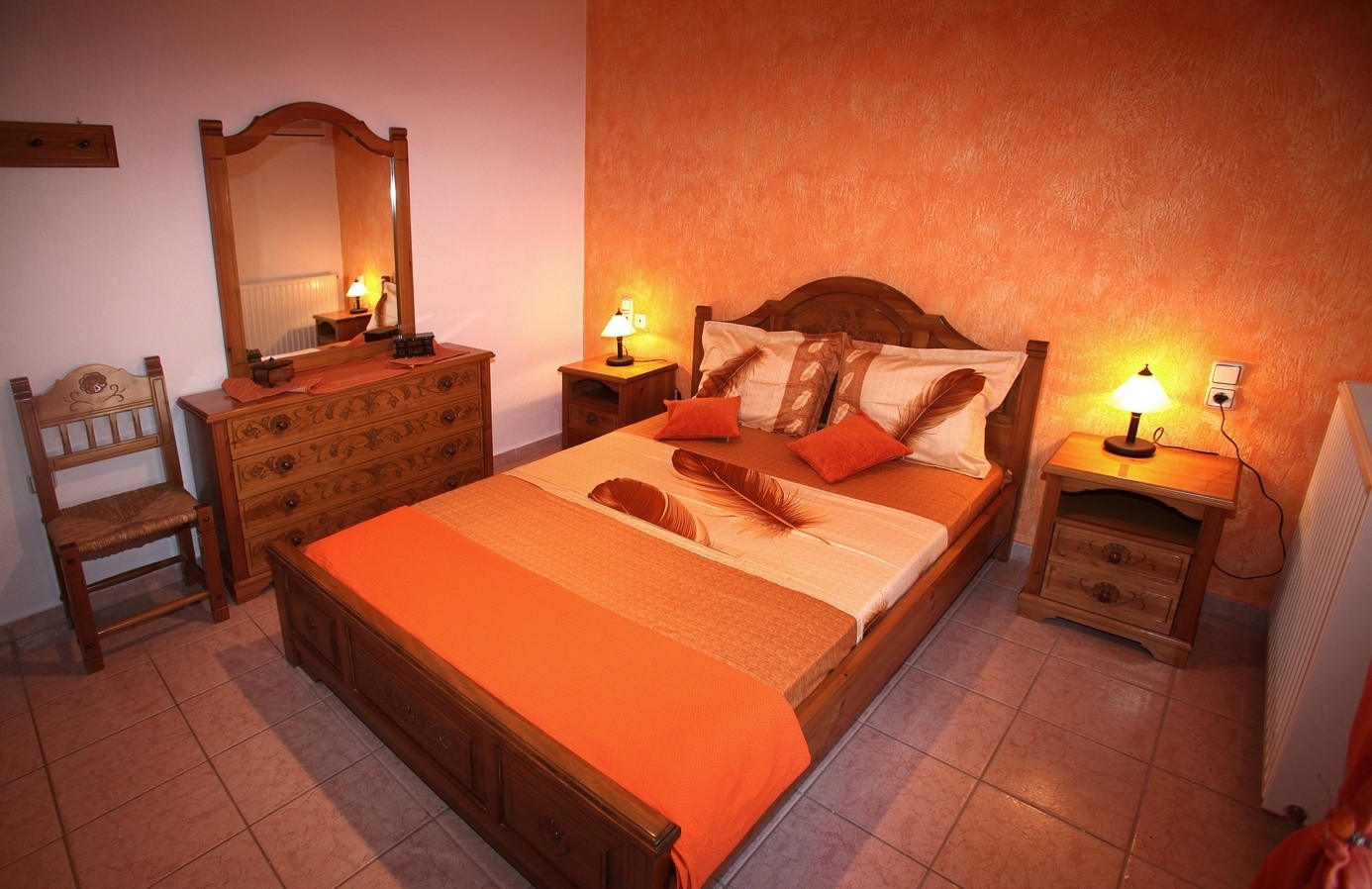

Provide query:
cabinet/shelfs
left=176, top=342, right=494, bottom=606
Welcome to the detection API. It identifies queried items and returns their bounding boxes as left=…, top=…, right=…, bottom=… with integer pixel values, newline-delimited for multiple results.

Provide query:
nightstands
left=557, top=352, right=679, bottom=450
left=1017, top=432, right=1243, bottom=669
left=312, top=309, right=373, bottom=346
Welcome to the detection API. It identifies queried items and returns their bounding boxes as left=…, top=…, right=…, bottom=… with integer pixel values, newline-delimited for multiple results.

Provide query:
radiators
left=239, top=274, right=339, bottom=357
left=1261, top=379, right=1372, bottom=829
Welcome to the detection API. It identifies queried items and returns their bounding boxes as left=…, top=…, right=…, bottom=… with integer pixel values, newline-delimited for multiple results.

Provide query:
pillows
left=828, top=341, right=1028, bottom=480
left=652, top=395, right=741, bottom=439
left=692, top=321, right=847, bottom=438
left=786, top=414, right=913, bottom=483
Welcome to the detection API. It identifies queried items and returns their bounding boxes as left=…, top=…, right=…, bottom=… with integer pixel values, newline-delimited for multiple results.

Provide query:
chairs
left=10, top=356, right=230, bottom=675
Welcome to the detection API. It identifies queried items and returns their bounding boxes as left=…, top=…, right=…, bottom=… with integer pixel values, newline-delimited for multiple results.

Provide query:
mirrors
left=198, top=101, right=417, bottom=381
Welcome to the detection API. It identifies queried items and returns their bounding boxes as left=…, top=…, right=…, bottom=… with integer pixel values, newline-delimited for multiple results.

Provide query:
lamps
left=1104, top=363, right=1173, bottom=457
left=345, top=279, right=368, bottom=314
left=601, top=308, right=637, bottom=366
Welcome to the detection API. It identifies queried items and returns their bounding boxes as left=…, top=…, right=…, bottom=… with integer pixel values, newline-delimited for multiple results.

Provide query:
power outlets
left=1204, top=363, right=1242, bottom=409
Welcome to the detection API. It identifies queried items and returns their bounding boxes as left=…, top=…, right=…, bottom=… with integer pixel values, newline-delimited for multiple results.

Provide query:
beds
left=260, top=277, right=1048, bottom=889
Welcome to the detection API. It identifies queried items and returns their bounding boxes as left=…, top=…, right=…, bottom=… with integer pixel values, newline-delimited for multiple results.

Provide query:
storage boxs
left=393, top=332, right=435, bottom=358
left=252, top=356, right=294, bottom=387
